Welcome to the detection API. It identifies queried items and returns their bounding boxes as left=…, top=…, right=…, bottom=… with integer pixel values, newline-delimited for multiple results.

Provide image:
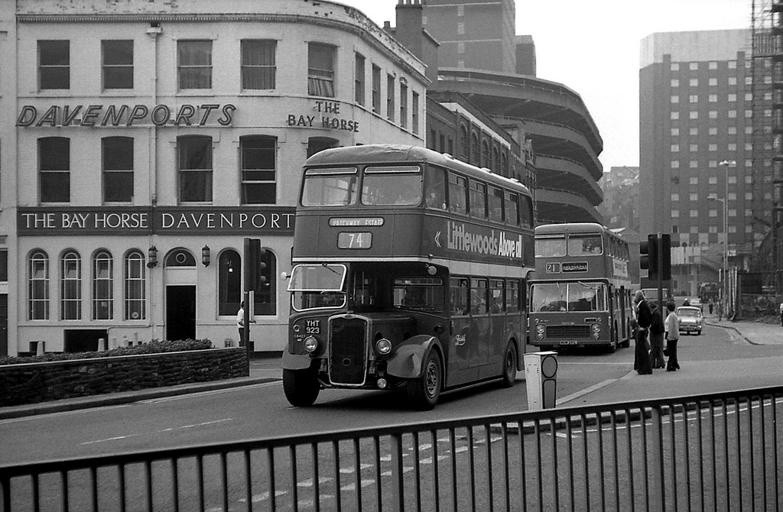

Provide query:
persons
left=664, top=301, right=680, bottom=371
left=648, top=301, right=664, bottom=368
left=237, top=301, right=256, bottom=346
left=682, top=298, right=690, bottom=306
left=634, top=291, right=652, bottom=374
left=708, top=299, right=714, bottom=314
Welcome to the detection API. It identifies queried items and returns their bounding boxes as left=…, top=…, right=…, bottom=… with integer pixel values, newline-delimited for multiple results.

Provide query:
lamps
left=200, top=243, right=211, bottom=268
left=146, top=244, right=157, bottom=268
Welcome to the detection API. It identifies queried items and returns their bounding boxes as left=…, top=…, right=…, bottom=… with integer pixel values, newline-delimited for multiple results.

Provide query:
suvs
left=639, top=288, right=674, bottom=307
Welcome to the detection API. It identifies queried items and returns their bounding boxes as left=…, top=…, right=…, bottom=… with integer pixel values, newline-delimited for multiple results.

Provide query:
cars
left=672, top=305, right=704, bottom=335
left=688, top=297, right=702, bottom=312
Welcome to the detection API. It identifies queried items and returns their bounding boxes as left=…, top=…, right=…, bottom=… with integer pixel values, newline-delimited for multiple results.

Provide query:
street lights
left=243, top=237, right=267, bottom=291
left=706, top=195, right=727, bottom=314
left=718, top=159, right=736, bottom=315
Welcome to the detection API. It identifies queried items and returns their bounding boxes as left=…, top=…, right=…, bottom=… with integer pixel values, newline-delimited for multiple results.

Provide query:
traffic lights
left=638, top=232, right=656, bottom=280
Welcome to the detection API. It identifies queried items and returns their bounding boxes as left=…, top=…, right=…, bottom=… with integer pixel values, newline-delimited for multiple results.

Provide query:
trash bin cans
left=524, top=351, right=558, bottom=410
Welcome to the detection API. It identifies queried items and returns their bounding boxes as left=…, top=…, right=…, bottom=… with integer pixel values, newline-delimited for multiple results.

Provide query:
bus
left=528, top=217, right=634, bottom=355
left=275, top=143, right=534, bottom=409
